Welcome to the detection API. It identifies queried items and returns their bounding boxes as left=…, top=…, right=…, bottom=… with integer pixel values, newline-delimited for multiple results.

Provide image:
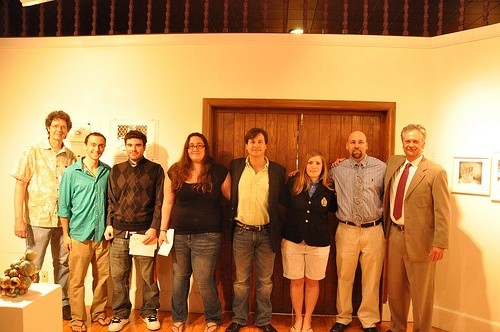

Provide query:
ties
left=393, top=163, right=412, bottom=220
left=351, top=163, right=364, bottom=227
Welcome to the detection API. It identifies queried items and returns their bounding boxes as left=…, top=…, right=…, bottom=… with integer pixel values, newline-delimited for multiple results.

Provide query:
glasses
left=51, top=125, right=68, bottom=130
left=187, top=144, right=205, bottom=150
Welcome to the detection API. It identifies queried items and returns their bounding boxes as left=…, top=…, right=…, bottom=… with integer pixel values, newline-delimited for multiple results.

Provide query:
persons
left=288, top=130, right=387, bottom=332
left=329, top=123, right=451, bottom=332
left=12, top=112, right=81, bottom=320
left=278, top=148, right=342, bottom=332
left=100, top=129, right=163, bottom=331
left=156, top=132, right=231, bottom=332
left=55, top=131, right=112, bottom=332
left=223, top=128, right=287, bottom=332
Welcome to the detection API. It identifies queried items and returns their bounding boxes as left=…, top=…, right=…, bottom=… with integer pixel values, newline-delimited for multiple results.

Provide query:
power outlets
left=42, top=271, right=48, bottom=282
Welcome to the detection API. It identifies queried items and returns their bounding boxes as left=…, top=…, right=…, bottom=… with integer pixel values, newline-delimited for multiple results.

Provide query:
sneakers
left=143, top=315, right=160, bottom=330
left=108, top=317, right=129, bottom=331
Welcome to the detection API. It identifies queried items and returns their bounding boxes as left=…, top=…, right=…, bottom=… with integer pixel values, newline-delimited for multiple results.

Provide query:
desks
left=0, top=282, right=64, bottom=332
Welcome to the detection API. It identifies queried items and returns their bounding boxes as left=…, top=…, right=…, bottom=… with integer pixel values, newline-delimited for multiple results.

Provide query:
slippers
left=204, top=321, right=217, bottom=332
left=97, top=316, right=111, bottom=326
left=71, top=322, right=87, bottom=332
left=171, top=321, right=185, bottom=332
left=290, top=323, right=313, bottom=332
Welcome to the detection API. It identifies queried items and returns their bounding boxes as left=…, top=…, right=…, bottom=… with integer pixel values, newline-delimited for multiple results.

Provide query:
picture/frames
left=449, top=156, right=490, bottom=196
left=490, top=152, right=500, bottom=202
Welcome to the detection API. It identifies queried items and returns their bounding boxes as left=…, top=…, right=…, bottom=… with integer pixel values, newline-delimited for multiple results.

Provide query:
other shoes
left=63, top=305, right=72, bottom=320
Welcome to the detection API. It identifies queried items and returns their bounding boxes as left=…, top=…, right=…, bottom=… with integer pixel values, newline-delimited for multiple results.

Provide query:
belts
left=237, top=222, right=270, bottom=231
left=392, top=221, right=405, bottom=231
left=339, top=218, right=382, bottom=228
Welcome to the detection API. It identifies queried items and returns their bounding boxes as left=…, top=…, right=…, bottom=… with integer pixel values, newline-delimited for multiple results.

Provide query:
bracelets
left=159, top=230, right=167, bottom=232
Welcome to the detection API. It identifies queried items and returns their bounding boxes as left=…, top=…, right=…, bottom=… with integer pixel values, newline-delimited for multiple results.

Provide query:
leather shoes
left=361, top=324, right=377, bottom=332
left=255, top=324, right=278, bottom=332
left=330, top=323, right=351, bottom=332
left=225, top=322, right=247, bottom=332
